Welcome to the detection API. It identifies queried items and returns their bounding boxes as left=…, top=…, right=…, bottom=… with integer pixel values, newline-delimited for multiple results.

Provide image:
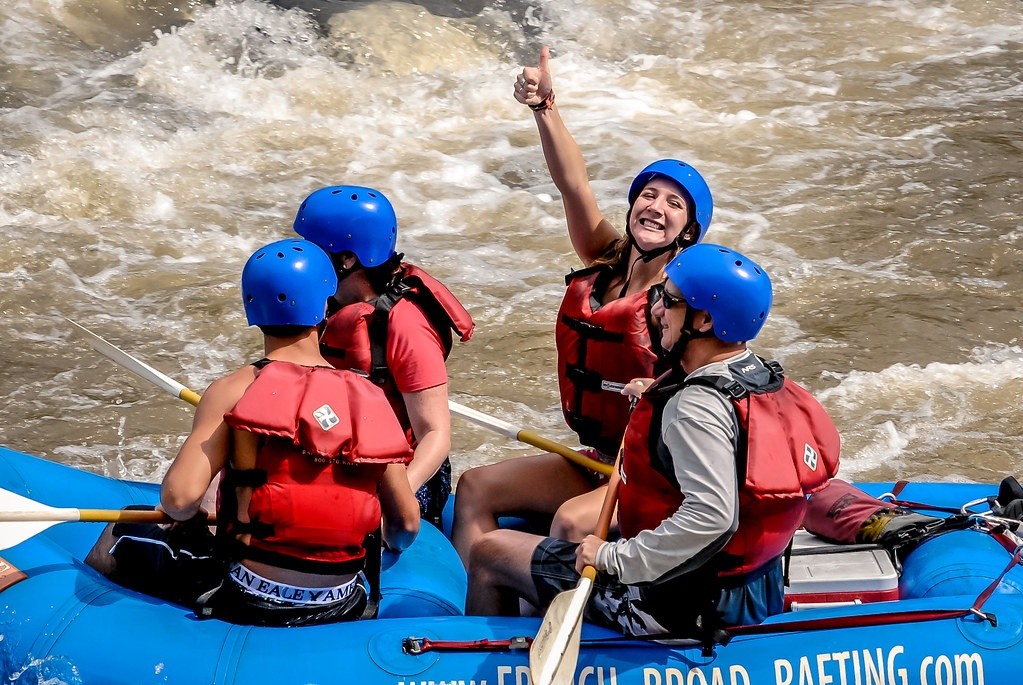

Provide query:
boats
left=0, top=444, right=1021, bottom=684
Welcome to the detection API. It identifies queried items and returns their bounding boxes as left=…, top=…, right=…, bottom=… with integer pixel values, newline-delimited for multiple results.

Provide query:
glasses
left=660, top=288, right=687, bottom=309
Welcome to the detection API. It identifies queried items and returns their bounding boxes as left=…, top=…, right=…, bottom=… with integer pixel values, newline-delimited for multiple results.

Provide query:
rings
left=518, top=88, right=523, bottom=93
left=520, top=79, right=526, bottom=85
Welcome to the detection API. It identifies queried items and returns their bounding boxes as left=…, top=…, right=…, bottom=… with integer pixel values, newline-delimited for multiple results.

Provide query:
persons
left=453, top=45, right=713, bottom=573
left=293, top=185, right=476, bottom=525
left=464, top=243, right=841, bottom=644
left=155, top=239, right=420, bottom=629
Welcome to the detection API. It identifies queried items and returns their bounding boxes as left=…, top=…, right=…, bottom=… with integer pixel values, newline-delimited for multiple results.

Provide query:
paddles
left=0, top=486, right=167, bottom=554
left=75, top=316, right=195, bottom=410
left=529, top=421, right=630, bottom=685
left=448, top=395, right=614, bottom=476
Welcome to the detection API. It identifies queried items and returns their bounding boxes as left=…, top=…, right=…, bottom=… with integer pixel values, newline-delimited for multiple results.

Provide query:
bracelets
left=528, top=89, right=555, bottom=112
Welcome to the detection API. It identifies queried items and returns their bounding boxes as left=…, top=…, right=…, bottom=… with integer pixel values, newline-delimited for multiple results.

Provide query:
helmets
left=664, top=243, right=773, bottom=343
left=628, top=159, right=713, bottom=247
left=242, top=238, right=338, bottom=327
left=293, top=185, right=397, bottom=267
